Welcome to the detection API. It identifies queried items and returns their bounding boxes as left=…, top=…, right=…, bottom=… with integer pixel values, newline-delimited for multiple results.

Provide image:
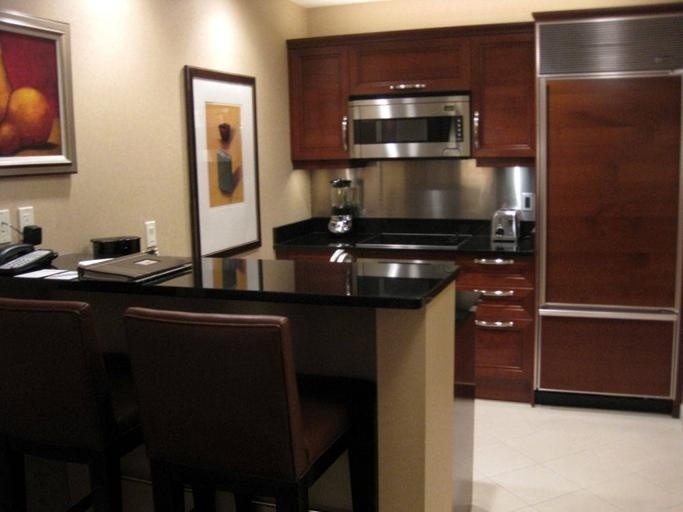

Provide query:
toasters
left=490, top=241, right=520, bottom=251
left=490, top=207, right=522, bottom=242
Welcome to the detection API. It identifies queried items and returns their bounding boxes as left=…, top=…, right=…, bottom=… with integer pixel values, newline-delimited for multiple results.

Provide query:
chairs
left=0, top=298, right=144, bottom=512
left=125, top=304, right=372, bottom=511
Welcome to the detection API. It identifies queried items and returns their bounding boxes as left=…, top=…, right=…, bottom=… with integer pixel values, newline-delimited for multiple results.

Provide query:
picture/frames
left=189, top=258, right=266, bottom=291
left=182, top=65, right=263, bottom=258
left=0, top=10, right=82, bottom=182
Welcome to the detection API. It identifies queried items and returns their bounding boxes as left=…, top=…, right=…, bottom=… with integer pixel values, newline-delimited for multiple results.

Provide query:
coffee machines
left=327, top=177, right=357, bottom=242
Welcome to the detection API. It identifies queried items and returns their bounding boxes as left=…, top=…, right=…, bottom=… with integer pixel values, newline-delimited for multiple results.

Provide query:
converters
left=23, top=226, right=42, bottom=246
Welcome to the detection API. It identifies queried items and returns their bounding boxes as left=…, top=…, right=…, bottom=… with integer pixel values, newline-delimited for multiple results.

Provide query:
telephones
left=1, top=244, right=59, bottom=274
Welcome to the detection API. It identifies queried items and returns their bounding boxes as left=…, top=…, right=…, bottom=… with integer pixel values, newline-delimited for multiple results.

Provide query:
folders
left=77, top=251, right=193, bottom=283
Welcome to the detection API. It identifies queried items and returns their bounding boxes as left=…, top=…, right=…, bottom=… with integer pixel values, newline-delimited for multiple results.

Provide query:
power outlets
left=15, top=206, right=34, bottom=241
left=0, top=206, right=14, bottom=249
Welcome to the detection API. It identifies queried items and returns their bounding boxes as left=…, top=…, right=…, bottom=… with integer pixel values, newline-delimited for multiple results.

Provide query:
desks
left=3, top=253, right=464, bottom=511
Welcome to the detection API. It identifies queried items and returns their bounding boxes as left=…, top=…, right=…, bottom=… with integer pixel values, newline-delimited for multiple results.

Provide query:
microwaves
left=348, top=259, right=460, bottom=303
left=346, top=95, right=469, bottom=162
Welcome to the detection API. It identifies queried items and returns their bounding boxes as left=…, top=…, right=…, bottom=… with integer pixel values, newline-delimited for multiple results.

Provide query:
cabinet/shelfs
left=271, top=240, right=355, bottom=263
left=351, top=25, right=472, bottom=94
left=526, top=1, right=682, bottom=419
left=445, top=245, right=538, bottom=408
left=468, top=18, right=537, bottom=161
left=285, top=34, right=351, bottom=162
